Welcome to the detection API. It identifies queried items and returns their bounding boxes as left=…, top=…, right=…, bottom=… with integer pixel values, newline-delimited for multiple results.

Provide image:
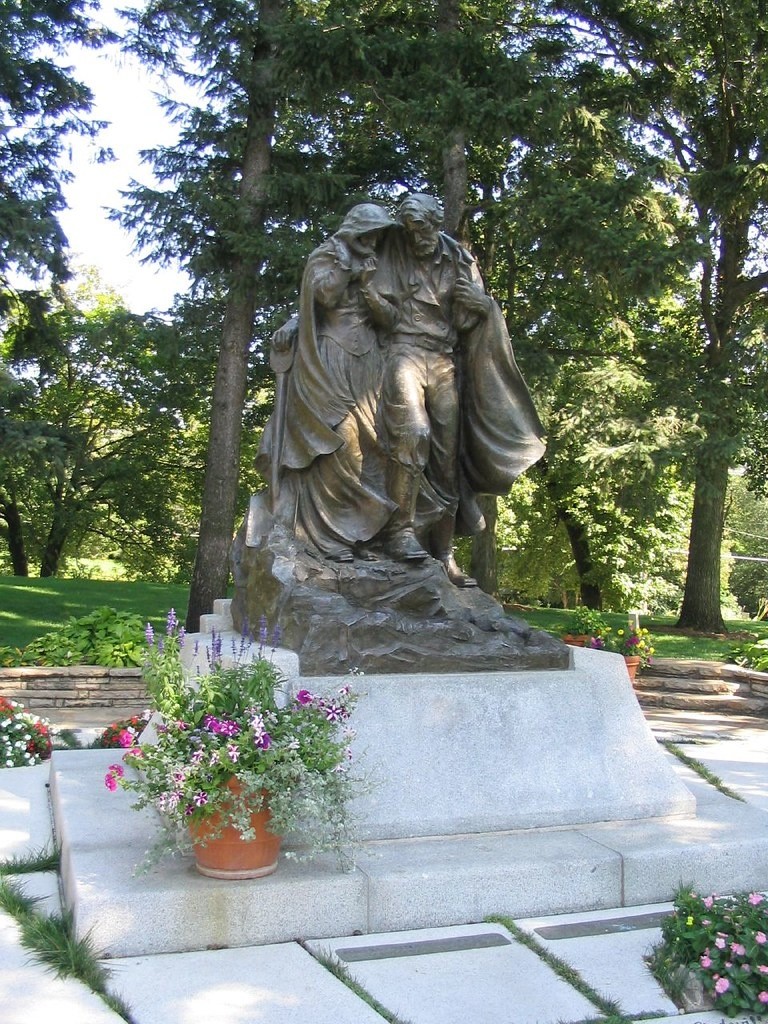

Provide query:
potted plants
left=560, top=605, right=606, bottom=648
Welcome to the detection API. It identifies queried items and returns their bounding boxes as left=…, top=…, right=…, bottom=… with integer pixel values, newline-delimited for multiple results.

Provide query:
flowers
left=90, top=708, right=158, bottom=749
left=648, top=874, right=768, bottom=1019
left=0, top=695, right=53, bottom=768
left=585, top=605, right=655, bottom=669
left=97, top=607, right=360, bottom=880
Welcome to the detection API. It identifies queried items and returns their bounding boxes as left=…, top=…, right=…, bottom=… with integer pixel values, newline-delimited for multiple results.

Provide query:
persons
left=253, top=193, right=549, bottom=586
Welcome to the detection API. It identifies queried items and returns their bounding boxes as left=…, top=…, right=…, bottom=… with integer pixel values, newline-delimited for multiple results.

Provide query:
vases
left=624, top=656, right=640, bottom=687
left=192, top=774, right=282, bottom=879
